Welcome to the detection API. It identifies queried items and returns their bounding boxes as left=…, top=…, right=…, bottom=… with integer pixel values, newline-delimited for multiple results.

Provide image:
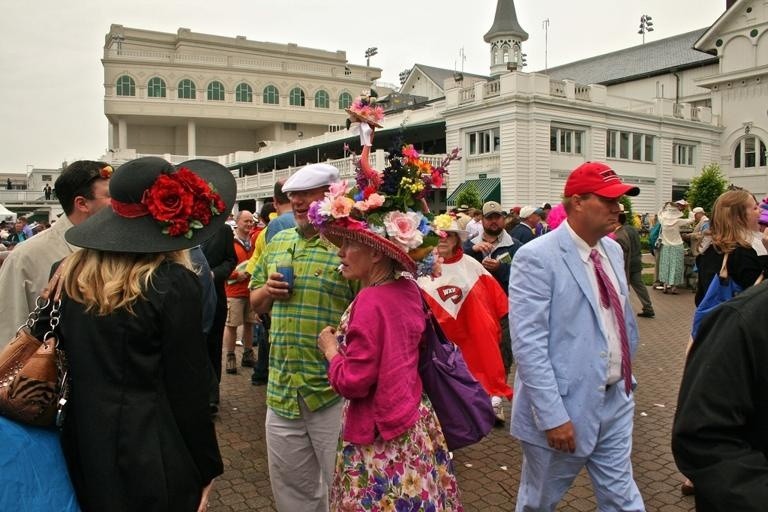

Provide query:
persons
left=0, top=124, right=768, bottom=512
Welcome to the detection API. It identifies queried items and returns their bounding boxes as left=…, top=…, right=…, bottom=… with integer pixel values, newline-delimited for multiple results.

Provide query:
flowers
left=141, top=168, right=224, bottom=239
left=350, top=95, right=385, bottom=123
left=309, top=140, right=461, bottom=278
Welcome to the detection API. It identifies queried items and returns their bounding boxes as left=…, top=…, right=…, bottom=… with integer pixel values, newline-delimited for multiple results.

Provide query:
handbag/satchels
left=0, top=330, right=66, bottom=425
left=418, top=343, right=494, bottom=452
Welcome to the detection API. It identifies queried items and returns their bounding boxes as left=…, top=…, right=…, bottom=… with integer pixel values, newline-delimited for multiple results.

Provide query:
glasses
left=244, top=240, right=250, bottom=251
left=82, top=162, right=113, bottom=187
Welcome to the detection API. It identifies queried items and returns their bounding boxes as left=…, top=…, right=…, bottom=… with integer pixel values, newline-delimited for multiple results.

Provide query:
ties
left=591, top=250, right=631, bottom=399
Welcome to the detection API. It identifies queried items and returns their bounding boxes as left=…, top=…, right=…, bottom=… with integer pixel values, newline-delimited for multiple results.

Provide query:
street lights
left=363, top=48, right=378, bottom=65
left=637, top=14, right=654, bottom=45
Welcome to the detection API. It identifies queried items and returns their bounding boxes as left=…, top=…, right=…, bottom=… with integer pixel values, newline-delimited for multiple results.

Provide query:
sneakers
left=637, top=313, right=655, bottom=317
left=225, top=352, right=239, bottom=374
left=243, top=350, right=257, bottom=365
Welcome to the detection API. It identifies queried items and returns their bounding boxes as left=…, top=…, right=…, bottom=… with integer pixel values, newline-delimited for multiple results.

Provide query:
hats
left=282, top=163, right=339, bottom=192
left=481, top=201, right=503, bottom=219
left=662, top=207, right=683, bottom=219
left=65, top=158, right=236, bottom=253
left=431, top=216, right=469, bottom=240
left=692, top=207, right=703, bottom=215
left=519, top=206, right=542, bottom=220
left=321, top=220, right=422, bottom=280
left=538, top=202, right=552, bottom=210
left=564, top=162, right=640, bottom=200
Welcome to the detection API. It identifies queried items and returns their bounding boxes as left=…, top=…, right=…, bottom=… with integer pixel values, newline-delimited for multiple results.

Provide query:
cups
left=275, top=257, right=295, bottom=296
